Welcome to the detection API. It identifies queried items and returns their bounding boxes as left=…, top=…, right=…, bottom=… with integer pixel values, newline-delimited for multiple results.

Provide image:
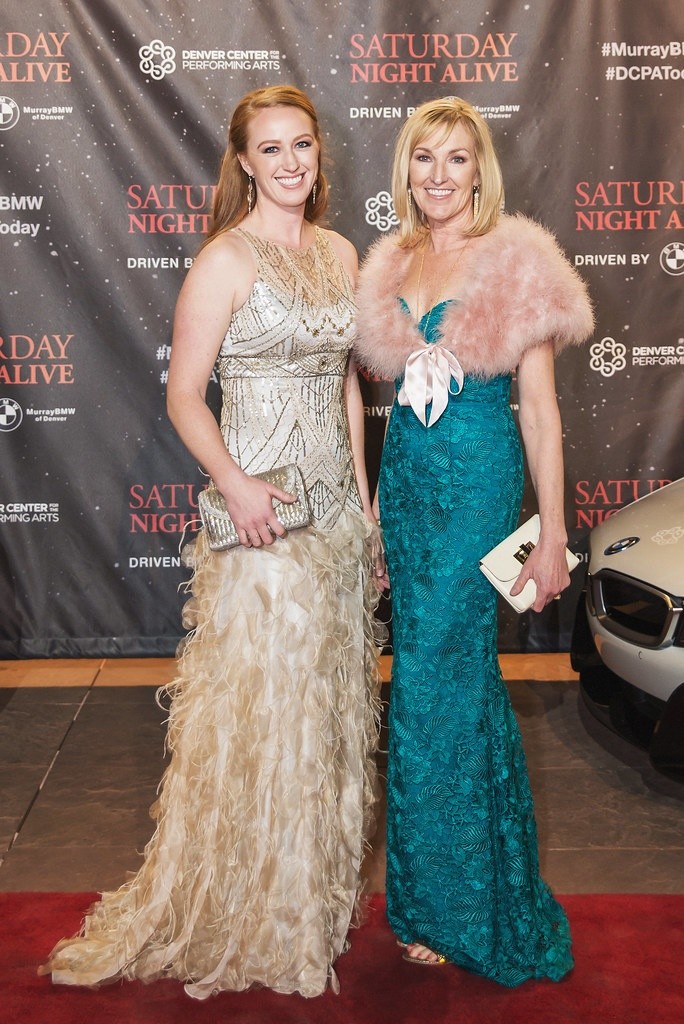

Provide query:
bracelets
left=375, top=519, right=381, bottom=525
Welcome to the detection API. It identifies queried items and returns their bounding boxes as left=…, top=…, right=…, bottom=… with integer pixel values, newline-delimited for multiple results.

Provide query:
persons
left=353, top=96, right=572, bottom=964
left=164, top=85, right=381, bottom=989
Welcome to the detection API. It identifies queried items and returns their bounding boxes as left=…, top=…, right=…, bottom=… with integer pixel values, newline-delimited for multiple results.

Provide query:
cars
left=581, top=478, right=684, bottom=705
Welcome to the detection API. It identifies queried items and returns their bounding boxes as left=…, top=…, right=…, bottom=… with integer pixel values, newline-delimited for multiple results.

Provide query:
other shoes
left=396, top=937, right=454, bottom=964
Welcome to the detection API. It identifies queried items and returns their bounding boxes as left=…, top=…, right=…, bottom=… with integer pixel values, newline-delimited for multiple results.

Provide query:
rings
left=247, top=533, right=260, bottom=541
left=553, top=594, right=561, bottom=600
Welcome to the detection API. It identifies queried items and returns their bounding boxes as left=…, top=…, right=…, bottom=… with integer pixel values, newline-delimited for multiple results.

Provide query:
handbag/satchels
left=479, top=513, right=580, bottom=614
left=197, top=464, right=311, bottom=551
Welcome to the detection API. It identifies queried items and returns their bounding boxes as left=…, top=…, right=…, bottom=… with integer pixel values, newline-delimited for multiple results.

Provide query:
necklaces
left=416, top=232, right=472, bottom=339
